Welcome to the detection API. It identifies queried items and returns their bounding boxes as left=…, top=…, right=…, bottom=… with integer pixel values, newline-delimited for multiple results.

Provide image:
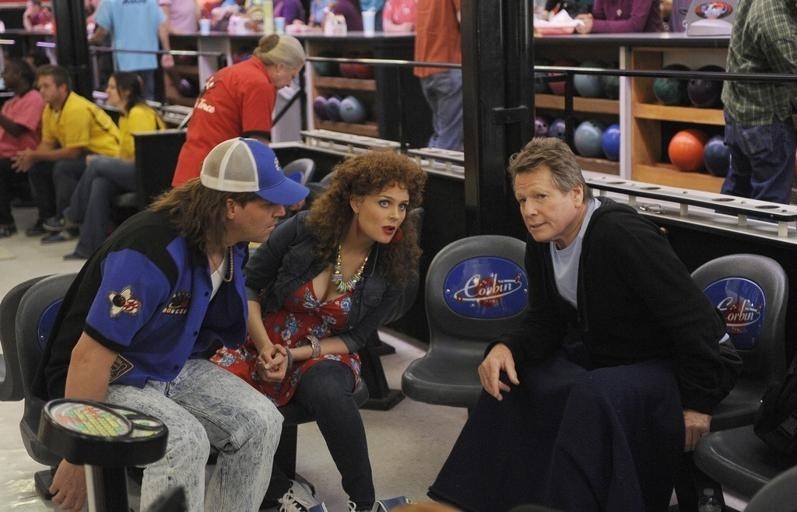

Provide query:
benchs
left=1, top=91, right=194, bottom=213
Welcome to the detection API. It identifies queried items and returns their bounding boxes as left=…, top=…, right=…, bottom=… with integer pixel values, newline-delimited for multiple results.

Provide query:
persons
left=714, top=0, right=797, bottom=223
left=171, top=34, right=306, bottom=190
left=209, top=147, right=424, bottom=512
left=390, top=137, right=746, bottom=512
left=380, top=2, right=414, bottom=33
left=574, top=0, right=664, bottom=34
left=271, top=1, right=367, bottom=30
left=161, top=0, right=200, bottom=62
left=89, top=1, right=174, bottom=105
left=1, top=61, right=166, bottom=258
left=33, top=136, right=310, bottom=512
left=23, top=2, right=51, bottom=32
left=413, top=0, right=465, bottom=153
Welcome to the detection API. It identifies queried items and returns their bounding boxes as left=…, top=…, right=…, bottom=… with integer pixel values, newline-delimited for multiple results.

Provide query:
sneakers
left=26, top=214, right=72, bottom=243
left=278, top=479, right=325, bottom=511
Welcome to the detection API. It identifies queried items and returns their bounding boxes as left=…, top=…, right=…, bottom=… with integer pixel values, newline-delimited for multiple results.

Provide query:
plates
left=535, top=25, right=575, bottom=36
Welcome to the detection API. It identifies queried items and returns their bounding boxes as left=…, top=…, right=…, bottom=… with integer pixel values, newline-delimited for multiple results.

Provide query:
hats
left=200, top=137, right=310, bottom=205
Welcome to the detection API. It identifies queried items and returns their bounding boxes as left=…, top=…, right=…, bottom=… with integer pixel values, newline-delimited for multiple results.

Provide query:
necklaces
left=331, top=244, right=369, bottom=294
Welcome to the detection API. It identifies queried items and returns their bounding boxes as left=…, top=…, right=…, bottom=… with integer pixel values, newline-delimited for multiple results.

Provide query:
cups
left=199, top=18, right=212, bottom=36
left=334, top=24, right=346, bottom=35
left=361, top=10, right=376, bottom=35
left=273, top=16, right=286, bottom=34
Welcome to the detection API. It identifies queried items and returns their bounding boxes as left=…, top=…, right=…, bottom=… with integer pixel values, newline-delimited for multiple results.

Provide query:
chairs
left=13, top=272, right=81, bottom=500
left=743, top=466, right=796, bottom=511
left=282, top=157, right=316, bottom=186
left=666, top=251, right=790, bottom=511
left=1, top=275, right=59, bottom=402
left=400, top=234, right=528, bottom=421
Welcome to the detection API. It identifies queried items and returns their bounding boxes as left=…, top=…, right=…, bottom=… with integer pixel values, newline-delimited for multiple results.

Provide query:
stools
left=274, top=377, right=370, bottom=499
left=694, top=356, right=796, bottom=502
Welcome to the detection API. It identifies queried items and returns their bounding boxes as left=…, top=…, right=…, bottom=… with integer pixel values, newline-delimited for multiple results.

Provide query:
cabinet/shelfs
left=162, top=35, right=730, bottom=195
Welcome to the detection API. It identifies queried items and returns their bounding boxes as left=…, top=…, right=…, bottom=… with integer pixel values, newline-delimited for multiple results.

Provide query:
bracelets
left=285, top=347, right=293, bottom=372
left=301, top=335, right=320, bottom=357
